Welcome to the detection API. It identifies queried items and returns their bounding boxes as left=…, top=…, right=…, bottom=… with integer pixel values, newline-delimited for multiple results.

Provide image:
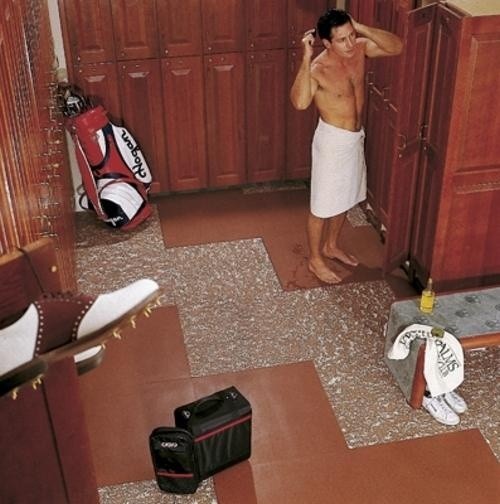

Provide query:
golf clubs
left=55, top=68, right=97, bottom=117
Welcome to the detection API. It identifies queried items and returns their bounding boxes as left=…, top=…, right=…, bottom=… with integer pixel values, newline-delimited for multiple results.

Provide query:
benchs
left=384, top=284, right=500, bottom=408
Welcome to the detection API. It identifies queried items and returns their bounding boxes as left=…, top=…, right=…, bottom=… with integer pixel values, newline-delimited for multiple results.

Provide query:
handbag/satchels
left=62, top=104, right=154, bottom=233
left=148, top=385, right=252, bottom=495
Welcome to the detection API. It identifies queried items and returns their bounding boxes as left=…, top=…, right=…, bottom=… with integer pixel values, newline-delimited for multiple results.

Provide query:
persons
left=290, top=8, right=403, bottom=284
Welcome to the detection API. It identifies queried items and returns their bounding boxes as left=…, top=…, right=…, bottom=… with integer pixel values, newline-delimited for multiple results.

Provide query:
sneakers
left=421, top=390, right=468, bottom=426
left=0, top=280, right=163, bottom=398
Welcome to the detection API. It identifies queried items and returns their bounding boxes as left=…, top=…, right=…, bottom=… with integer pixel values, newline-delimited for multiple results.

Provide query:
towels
left=387, top=322, right=465, bottom=398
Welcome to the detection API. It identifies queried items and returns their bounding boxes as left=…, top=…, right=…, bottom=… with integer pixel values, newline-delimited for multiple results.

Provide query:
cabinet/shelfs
left=243, top=0, right=287, bottom=185
left=285, top=2, right=328, bottom=180
left=156, top=0, right=208, bottom=192
left=61, top=0, right=122, bottom=128
left=0, top=0, right=77, bottom=299
left=108, top=0, right=165, bottom=195
left=201, top=1, right=249, bottom=191
left=359, top=2, right=458, bottom=291
